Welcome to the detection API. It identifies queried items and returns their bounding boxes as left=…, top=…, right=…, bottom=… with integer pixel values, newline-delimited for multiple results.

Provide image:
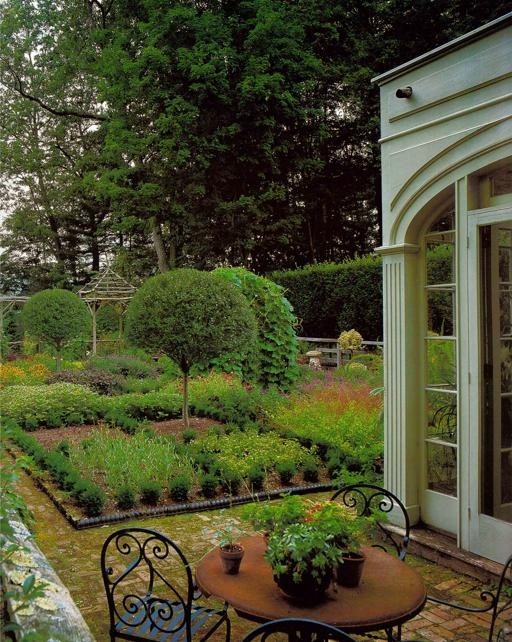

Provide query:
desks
left=195, top=535, right=429, bottom=641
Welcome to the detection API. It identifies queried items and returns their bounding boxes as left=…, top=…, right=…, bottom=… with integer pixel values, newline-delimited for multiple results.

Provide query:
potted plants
left=214, top=523, right=243, bottom=573
left=255, top=498, right=363, bottom=598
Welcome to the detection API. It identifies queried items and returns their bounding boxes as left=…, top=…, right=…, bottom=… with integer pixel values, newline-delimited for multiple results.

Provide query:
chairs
left=397, top=553, right=512, bottom=642
left=331, top=484, right=410, bottom=559
left=241, top=618, right=356, bottom=642
left=100, top=528, right=230, bottom=642
